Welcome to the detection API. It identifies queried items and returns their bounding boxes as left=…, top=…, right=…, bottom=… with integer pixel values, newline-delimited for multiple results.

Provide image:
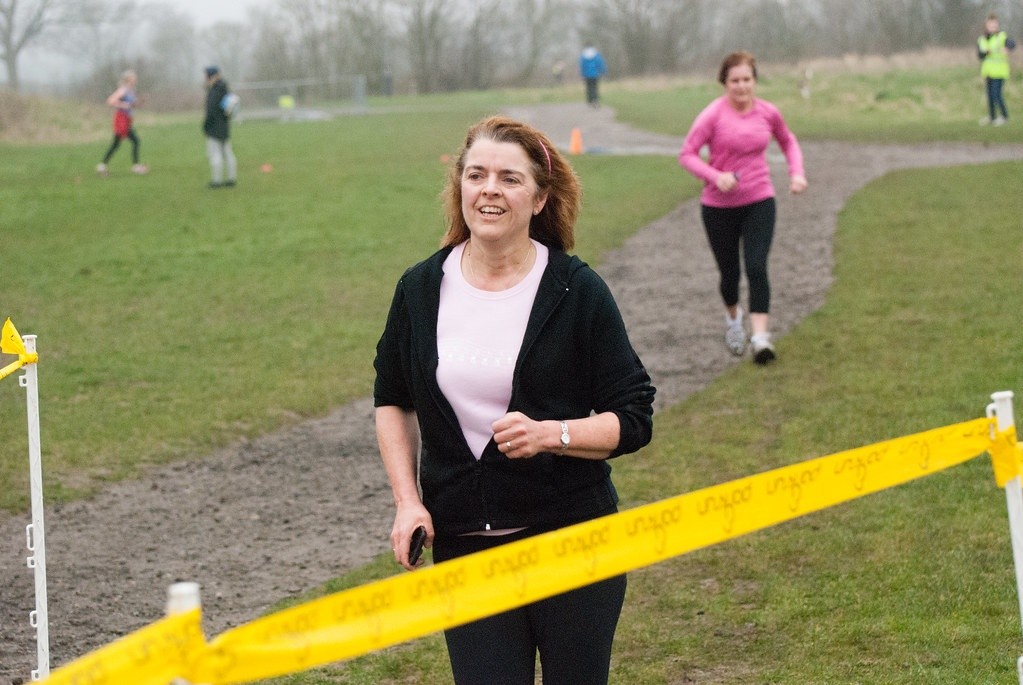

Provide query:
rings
left=507, top=442, right=512, bottom=452
left=392, top=549, right=396, bottom=552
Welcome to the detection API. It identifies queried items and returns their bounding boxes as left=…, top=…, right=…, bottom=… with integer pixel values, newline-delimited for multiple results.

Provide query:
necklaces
left=468, top=239, right=532, bottom=291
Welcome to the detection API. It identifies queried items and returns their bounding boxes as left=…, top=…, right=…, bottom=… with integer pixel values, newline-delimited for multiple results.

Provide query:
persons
left=976, top=15, right=1017, bottom=126
left=97, top=69, right=150, bottom=173
left=579, top=40, right=606, bottom=107
left=202, top=66, right=238, bottom=188
left=373, top=118, right=658, bottom=685
left=681, top=51, right=807, bottom=365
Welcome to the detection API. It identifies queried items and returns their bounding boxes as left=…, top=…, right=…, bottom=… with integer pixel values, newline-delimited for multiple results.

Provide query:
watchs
left=557, top=421, right=570, bottom=456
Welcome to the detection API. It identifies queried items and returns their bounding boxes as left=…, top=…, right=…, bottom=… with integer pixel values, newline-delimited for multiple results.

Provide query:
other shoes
left=980, top=116, right=994, bottom=126
left=724, top=307, right=749, bottom=354
left=992, top=115, right=1006, bottom=128
left=208, top=178, right=236, bottom=188
left=751, top=335, right=776, bottom=366
left=97, top=163, right=110, bottom=179
left=129, top=163, right=150, bottom=174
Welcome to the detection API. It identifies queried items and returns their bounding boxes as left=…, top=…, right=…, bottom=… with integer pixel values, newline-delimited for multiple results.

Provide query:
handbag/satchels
left=220, top=91, right=243, bottom=122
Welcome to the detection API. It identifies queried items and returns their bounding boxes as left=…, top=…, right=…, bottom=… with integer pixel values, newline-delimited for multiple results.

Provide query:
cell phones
left=408, top=526, right=426, bottom=566
